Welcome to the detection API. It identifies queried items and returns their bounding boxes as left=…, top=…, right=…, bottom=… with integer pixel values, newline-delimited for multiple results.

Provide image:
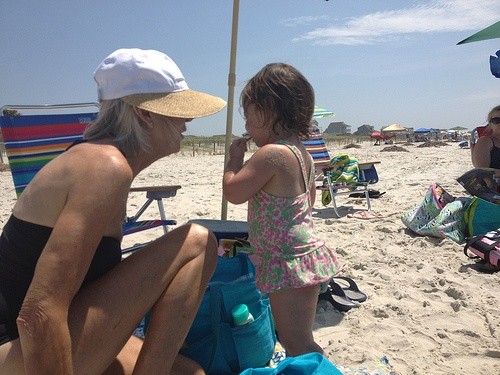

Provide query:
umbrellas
left=415, top=127, right=430, bottom=139
left=311, top=106, right=334, bottom=117
left=457, top=21, right=500, bottom=45
left=381, top=124, right=406, bottom=142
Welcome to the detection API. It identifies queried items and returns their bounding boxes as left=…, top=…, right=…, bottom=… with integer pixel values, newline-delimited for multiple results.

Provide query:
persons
left=224, top=63, right=341, bottom=360
left=0, top=48, right=229, bottom=375
left=472, top=106, right=500, bottom=168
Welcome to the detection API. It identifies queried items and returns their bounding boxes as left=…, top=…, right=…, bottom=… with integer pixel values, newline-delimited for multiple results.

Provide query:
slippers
left=318, top=277, right=367, bottom=311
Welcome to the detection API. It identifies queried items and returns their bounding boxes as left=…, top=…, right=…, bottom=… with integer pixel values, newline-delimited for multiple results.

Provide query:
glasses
left=489, top=117, right=500, bottom=124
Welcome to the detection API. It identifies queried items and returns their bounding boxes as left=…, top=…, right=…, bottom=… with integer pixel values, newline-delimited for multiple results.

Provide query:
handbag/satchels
left=401, top=182, right=500, bottom=244
left=143, top=248, right=277, bottom=375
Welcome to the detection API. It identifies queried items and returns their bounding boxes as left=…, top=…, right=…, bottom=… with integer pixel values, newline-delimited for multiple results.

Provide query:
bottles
left=230, top=304, right=254, bottom=327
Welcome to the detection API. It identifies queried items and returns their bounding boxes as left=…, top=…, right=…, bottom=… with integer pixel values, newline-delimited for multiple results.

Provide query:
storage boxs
left=189, top=219, right=249, bottom=255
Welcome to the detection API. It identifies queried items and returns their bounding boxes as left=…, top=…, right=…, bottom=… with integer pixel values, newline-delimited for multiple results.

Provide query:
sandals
left=360, top=189, right=380, bottom=198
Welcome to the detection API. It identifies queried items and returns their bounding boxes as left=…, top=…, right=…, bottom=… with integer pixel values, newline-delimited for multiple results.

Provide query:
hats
left=94, top=48, right=227, bottom=118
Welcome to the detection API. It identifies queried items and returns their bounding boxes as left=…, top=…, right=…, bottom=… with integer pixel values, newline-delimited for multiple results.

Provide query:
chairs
left=302, top=119, right=381, bottom=218
left=0, top=101, right=181, bottom=253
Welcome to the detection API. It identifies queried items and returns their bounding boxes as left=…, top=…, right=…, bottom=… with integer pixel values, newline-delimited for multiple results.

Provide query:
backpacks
left=463, top=228, right=500, bottom=267
